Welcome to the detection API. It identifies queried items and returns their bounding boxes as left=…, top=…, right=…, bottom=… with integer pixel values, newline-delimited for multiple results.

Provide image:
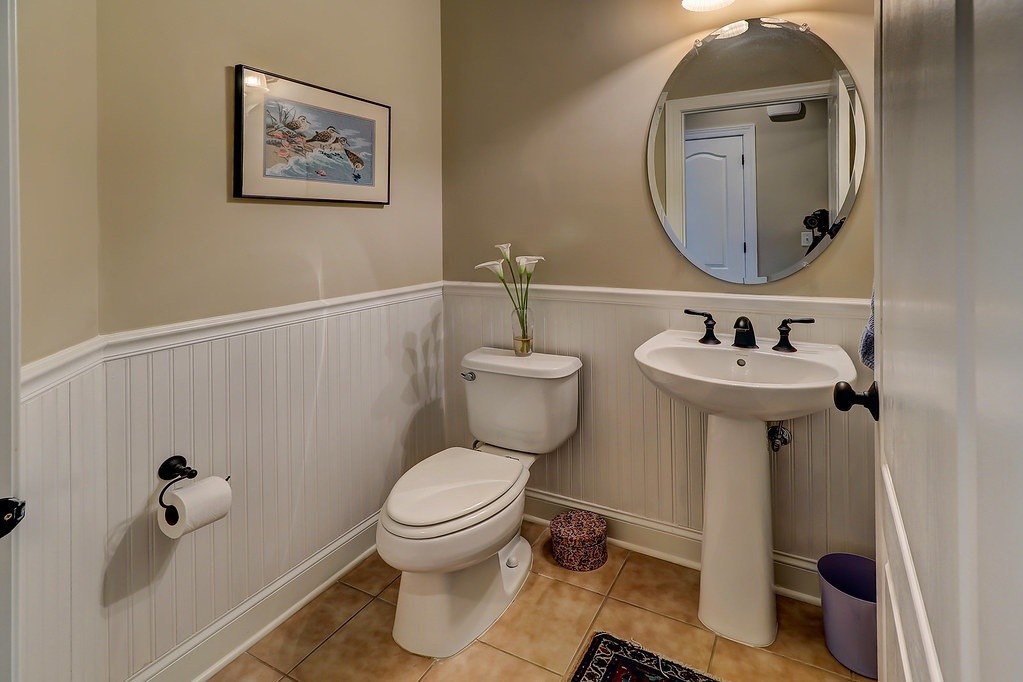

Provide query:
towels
left=858, top=280, right=875, bottom=372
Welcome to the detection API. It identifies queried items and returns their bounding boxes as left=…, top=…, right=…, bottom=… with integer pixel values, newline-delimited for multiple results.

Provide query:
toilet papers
left=156, top=475, right=232, bottom=540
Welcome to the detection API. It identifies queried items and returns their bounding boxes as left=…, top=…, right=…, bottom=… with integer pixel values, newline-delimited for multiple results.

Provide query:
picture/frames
left=235, top=64, right=391, bottom=206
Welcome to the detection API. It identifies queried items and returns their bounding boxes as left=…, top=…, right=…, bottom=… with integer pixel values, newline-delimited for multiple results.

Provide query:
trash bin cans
left=816, top=552, right=877, bottom=680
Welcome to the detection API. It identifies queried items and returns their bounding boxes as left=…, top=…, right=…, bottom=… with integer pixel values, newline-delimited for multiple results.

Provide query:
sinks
left=630, top=328, right=857, bottom=421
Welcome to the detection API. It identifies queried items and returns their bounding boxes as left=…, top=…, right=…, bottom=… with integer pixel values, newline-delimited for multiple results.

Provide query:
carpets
left=564, top=631, right=730, bottom=682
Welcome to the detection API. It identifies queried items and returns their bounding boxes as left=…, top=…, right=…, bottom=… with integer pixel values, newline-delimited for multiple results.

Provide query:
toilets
left=375, top=348, right=583, bottom=660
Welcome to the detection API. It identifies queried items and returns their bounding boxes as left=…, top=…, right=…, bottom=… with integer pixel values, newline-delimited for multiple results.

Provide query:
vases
left=511, top=306, right=534, bottom=357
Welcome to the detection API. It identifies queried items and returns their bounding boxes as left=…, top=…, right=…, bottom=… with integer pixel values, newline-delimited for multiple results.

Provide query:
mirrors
left=645, top=17, right=865, bottom=285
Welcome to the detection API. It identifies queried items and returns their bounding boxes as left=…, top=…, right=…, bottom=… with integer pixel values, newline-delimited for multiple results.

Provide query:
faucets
left=730, top=316, right=760, bottom=350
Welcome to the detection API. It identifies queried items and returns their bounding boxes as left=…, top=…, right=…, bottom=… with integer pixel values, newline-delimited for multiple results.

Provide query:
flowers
left=475, top=243, right=546, bottom=352
